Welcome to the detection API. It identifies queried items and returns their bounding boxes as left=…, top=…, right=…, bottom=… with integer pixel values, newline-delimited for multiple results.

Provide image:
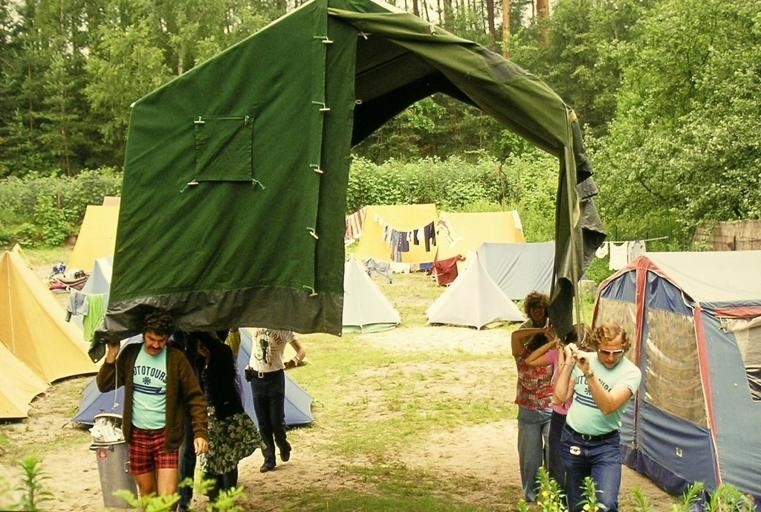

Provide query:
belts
left=566, top=425, right=617, bottom=441
left=253, top=369, right=283, bottom=378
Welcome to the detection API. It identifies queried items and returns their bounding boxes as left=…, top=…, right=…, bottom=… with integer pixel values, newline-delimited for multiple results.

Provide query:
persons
left=160, top=324, right=269, bottom=511
left=95, top=310, right=210, bottom=512
left=511, top=326, right=553, bottom=506
left=245, top=326, right=307, bottom=473
left=523, top=323, right=596, bottom=508
left=556, top=319, right=642, bottom=511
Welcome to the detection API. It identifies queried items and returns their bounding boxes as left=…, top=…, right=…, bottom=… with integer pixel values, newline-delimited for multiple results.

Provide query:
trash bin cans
left=89, top=413, right=138, bottom=509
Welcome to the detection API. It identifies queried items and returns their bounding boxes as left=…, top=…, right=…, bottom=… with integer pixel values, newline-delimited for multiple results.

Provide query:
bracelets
left=585, top=369, right=595, bottom=379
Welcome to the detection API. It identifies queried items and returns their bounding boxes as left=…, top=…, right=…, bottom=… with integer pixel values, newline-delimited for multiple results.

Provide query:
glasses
left=599, top=349, right=623, bottom=355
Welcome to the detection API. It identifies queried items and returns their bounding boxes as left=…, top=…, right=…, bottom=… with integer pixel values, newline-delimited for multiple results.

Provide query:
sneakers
left=280, top=444, right=291, bottom=461
left=261, top=463, right=276, bottom=472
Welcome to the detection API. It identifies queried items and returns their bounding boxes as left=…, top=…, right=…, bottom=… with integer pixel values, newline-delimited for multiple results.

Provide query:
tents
left=72, top=197, right=120, bottom=314
left=343, top=204, right=556, bottom=330
left=587, top=248, right=761, bottom=512
left=89, top=2, right=607, bottom=362
left=1, top=243, right=104, bottom=419
